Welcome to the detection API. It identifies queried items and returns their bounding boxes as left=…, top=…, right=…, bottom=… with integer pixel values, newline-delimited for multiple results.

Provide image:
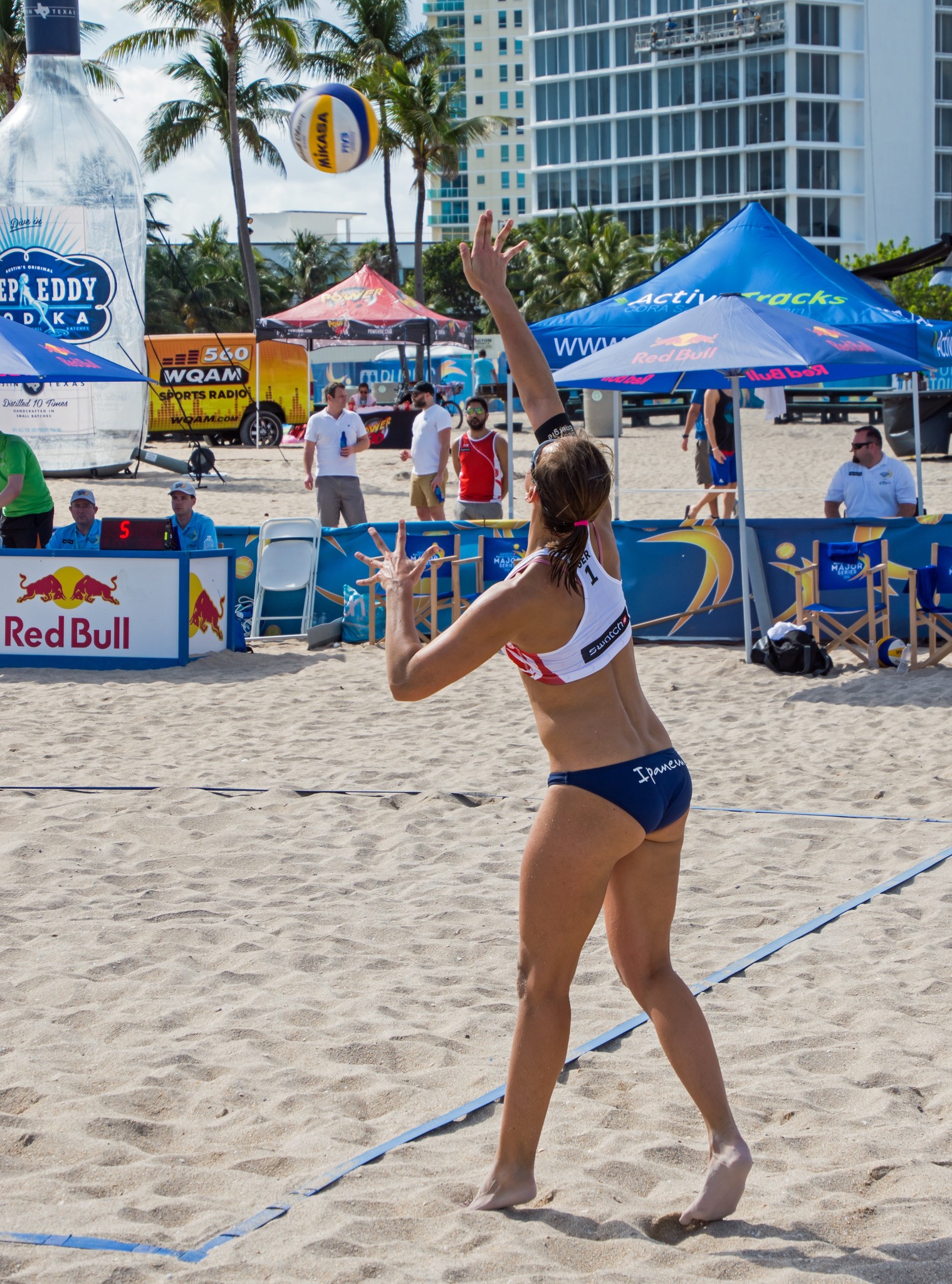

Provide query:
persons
left=0, top=430, right=54, bottom=549
left=162, top=481, right=219, bottom=551
left=683, top=389, right=738, bottom=519
left=451, top=397, right=514, bottom=521
left=400, top=380, right=451, bottom=521
left=44, top=489, right=101, bottom=550
left=824, top=426, right=917, bottom=518
left=349, top=382, right=376, bottom=408
left=682, top=389, right=739, bottom=520
left=304, top=382, right=370, bottom=528
left=471, top=349, right=498, bottom=411
left=393, top=381, right=418, bottom=410
left=354, top=206, right=751, bottom=1232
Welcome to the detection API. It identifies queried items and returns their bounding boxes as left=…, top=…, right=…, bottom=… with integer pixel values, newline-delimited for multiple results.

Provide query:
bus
left=142, top=332, right=310, bottom=449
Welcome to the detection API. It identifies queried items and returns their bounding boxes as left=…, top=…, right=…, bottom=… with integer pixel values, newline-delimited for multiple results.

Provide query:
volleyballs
left=875, top=636, right=906, bottom=668
left=290, top=83, right=379, bottom=173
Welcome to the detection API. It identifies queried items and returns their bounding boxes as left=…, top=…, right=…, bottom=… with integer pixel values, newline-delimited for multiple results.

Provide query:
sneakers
left=709, top=515, right=720, bottom=519
left=732, top=499, right=738, bottom=518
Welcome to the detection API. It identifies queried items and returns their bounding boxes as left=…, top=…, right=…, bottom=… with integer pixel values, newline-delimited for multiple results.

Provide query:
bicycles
left=394, top=375, right=464, bottom=430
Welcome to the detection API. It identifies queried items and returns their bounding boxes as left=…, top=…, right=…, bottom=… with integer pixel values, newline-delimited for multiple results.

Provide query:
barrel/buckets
left=583, top=387, right=622, bottom=437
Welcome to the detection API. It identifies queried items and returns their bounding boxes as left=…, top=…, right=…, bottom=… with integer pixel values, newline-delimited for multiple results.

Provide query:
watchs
left=682, top=433, right=689, bottom=438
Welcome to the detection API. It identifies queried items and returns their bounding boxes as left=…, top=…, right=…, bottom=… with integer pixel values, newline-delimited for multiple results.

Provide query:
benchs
left=783, top=400, right=883, bottom=425
left=565, top=397, right=699, bottom=427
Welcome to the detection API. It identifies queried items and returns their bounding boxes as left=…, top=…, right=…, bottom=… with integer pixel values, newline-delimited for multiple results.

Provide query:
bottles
left=0, top=0, right=151, bottom=475
left=340, top=431, right=347, bottom=456
left=312, top=613, right=318, bottom=627
left=867, top=640, right=879, bottom=669
left=897, top=644, right=912, bottom=674
left=430, top=478, right=445, bottom=503
left=203, top=535, right=214, bottom=550
left=318, top=612, right=327, bottom=625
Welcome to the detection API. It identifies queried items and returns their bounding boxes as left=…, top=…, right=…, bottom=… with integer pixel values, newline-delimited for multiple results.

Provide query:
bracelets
left=712, top=447, right=719, bottom=451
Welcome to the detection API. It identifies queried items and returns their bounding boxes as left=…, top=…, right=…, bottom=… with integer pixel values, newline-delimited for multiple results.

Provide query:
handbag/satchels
left=750, top=630, right=833, bottom=679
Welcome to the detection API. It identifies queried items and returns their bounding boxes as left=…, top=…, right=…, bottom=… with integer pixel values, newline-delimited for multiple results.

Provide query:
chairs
left=250, top=517, right=321, bottom=640
left=451, top=535, right=528, bottom=623
left=794, top=538, right=890, bottom=666
left=368, top=534, right=462, bottom=646
left=908, top=543, right=952, bottom=671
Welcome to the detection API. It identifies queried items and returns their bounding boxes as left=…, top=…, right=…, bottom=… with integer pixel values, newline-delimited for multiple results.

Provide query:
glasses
left=360, top=391, right=367, bottom=394
left=851, top=441, right=874, bottom=450
left=407, top=386, right=414, bottom=390
left=411, top=392, right=427, bottom=398
left=465, top=407, right=484, bottom=415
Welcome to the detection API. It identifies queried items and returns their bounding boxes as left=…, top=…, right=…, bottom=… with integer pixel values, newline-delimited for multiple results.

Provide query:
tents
left=254, top=263, right=481, bottom=458
left=505, top=202, right=952, bottom=521
left=550, top=294, right=937, bottom=522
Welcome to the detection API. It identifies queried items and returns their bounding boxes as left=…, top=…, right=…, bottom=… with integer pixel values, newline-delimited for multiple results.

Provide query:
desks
left=0, top=548, right=237, bottom=670
left=574, top=389, right=693, bottom=426
left=773, top=386, right=896, bottom=425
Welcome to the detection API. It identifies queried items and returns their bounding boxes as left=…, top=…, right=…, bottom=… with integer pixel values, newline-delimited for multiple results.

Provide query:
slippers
left=684, top=505, right=691, bottom=519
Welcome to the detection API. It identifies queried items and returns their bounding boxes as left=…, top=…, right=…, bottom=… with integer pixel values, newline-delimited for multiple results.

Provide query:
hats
left=465, top=397, right=488, bottom=413
left=70, top=489, right=95, bottom=506
left=406, top=381, right=435, bottom=397
left=167, top=481, right=197, bottom=498
